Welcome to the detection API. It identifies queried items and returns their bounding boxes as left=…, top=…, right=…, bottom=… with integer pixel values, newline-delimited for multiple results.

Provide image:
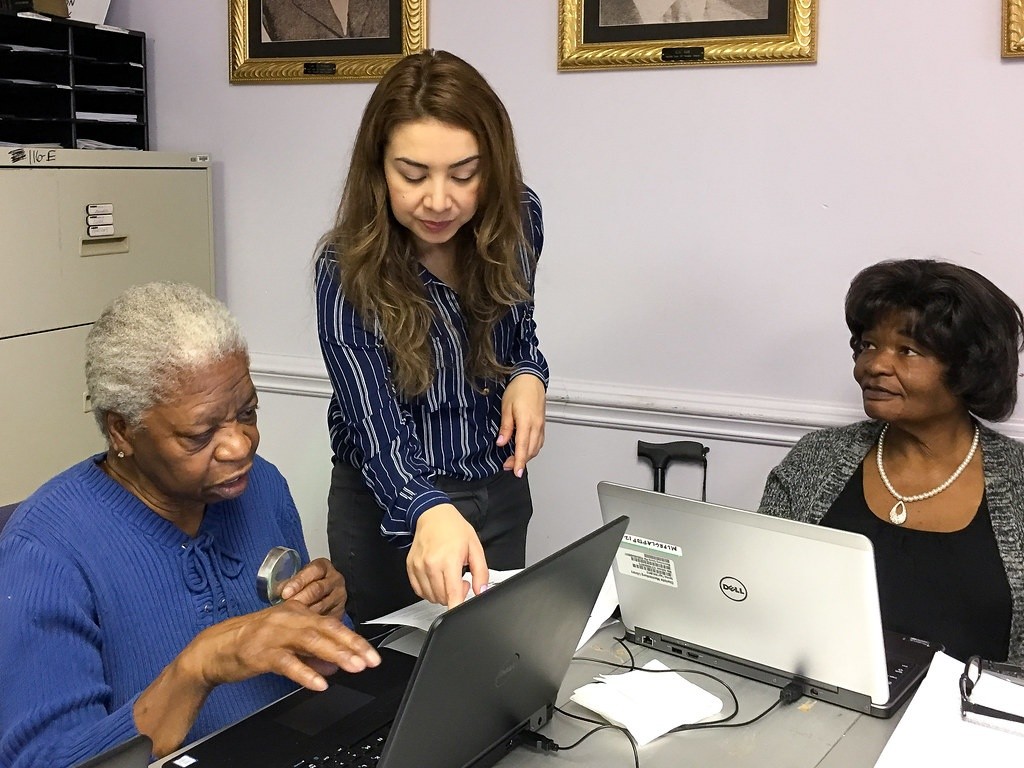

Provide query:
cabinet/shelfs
left=0, top=7, right=71, bottom=149
left=71, top=20, right=151, bottom=150
left=0, top=323, right=110, bottom=508
left=0, top=145, right=216, bottom=339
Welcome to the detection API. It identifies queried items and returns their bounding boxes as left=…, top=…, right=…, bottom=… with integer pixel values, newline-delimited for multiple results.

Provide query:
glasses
left=959, top=655, right=1024, bottom=722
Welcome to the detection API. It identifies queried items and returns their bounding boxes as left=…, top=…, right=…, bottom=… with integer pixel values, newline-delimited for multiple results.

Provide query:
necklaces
left=877, top=422, right=979, bottom=526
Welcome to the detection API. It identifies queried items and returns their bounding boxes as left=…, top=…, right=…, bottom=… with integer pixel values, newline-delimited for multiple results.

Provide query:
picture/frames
left=228, top=0, right=427, bottom=84
left=557, top=0, right=819, bottom=72
left=1002, top=0, right=1024, bottom=59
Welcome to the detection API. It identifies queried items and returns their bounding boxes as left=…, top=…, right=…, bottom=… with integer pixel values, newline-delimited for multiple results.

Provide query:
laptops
left=597, top=480, right=945, bottom=719
left=162, top=516, right=630, bottom=768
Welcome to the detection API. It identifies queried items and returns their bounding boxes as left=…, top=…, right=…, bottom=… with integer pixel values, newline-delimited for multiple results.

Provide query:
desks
left=148, top=617, right=1024, bottom=768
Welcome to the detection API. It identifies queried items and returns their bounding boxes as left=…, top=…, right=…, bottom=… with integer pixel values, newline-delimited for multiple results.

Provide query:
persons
left=315, top=49, right=549, bottom=640
left=0, top=280, right=382, bottom=768
left=757, top=260, right=1024, bottom=680
